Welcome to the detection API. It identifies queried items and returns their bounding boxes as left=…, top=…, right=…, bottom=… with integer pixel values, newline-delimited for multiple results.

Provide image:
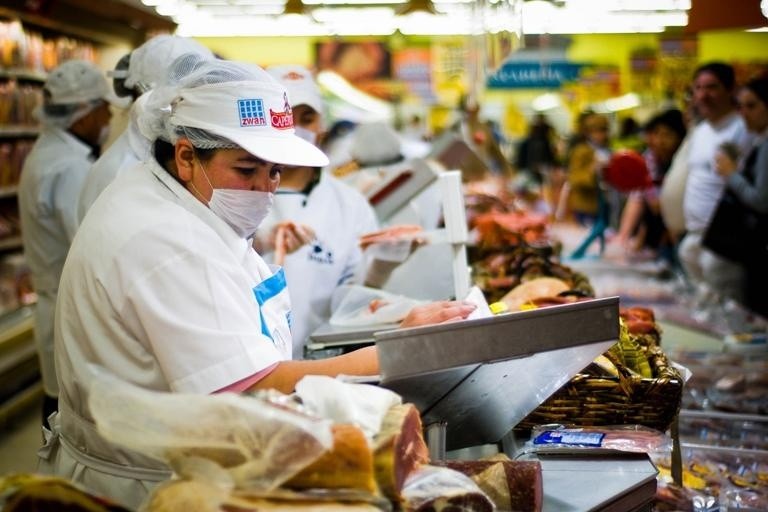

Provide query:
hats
left=41, top=60, right=133, bottom=110
left=266, top=65, right=325, bottom=118
left=170, top=78, right=330, bottom=167
left=125, top=34, right=204, bottom=85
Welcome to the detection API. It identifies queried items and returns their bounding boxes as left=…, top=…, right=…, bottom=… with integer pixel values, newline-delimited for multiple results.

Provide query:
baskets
left=439, top=191, right=682, bottom=437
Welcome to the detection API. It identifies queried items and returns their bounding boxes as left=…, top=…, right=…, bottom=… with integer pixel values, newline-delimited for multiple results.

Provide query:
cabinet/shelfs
left=1, top=4, right=132, bottom=345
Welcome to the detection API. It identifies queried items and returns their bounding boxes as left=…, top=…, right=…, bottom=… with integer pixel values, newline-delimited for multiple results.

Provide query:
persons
left=17, top=58, right=114, bottom=426
left=455, top=60, right=767, bottom=319
left=75, top=31, right=475, bottom=364
left=37, top=62, right=477, bottom=512
